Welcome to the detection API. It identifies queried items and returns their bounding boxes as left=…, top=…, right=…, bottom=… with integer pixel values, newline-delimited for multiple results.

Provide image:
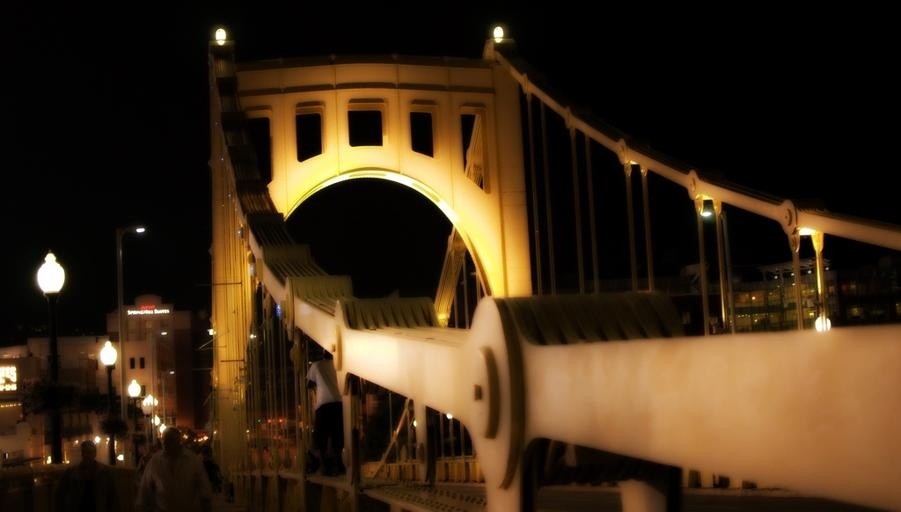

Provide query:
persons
left=53, top=440, right=118, bottom=511
left=307, top=346, right=347, bottom=476
left=136, top=426, right=221, bottom=511
